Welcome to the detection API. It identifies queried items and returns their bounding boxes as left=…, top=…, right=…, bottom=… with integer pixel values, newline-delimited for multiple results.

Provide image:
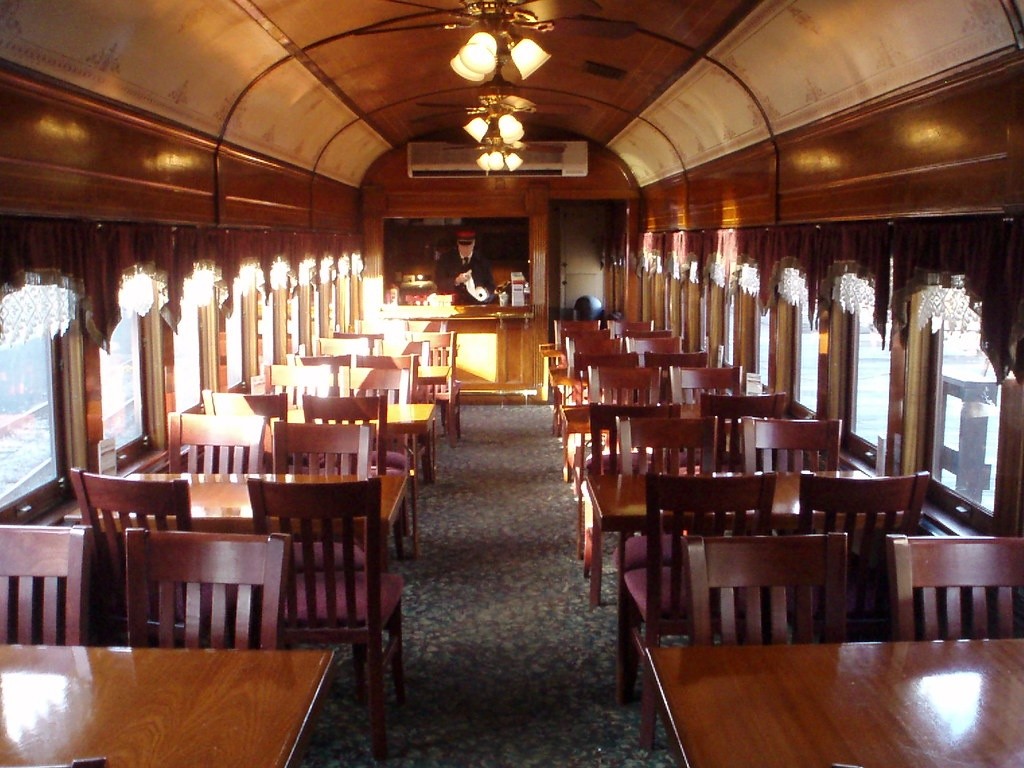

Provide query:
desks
left=270, top=404, right=438, bottom=561
left=62, top=473, right=409, bottom=574
left=0, top=644, right=339, bottom=767
left=538, top=343, right=1024, bottom=767
left=416, top=365, right=453, bottom=470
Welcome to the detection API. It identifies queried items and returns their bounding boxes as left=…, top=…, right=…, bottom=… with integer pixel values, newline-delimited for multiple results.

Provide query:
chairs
left=0, top=331, right=460, bottom=762
left=553, top=319, right=1024, bottom=751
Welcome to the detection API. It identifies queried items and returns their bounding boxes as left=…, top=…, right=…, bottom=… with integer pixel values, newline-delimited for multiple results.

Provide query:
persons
left=435, top=227, right=496, bottom=303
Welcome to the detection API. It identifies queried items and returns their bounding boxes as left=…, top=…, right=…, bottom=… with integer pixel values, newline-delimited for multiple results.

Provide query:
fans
left=409, top=82, right=593, bottom=125
left=350, top=0, right=638, bottom=40
left=441, top=130, right=565, bottom=154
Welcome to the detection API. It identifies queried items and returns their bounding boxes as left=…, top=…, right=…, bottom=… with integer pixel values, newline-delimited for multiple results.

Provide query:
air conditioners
left=406, top=142, right=589, bottom=179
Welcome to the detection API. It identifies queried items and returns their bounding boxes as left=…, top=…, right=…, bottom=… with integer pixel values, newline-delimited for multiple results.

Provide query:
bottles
left=391, top=284, right=398, bottom=306
left=347, top=322, right=353, bottom=332
left=499, top=291, right=509, bottom=307
left=524, top=283, right=530, bottom=305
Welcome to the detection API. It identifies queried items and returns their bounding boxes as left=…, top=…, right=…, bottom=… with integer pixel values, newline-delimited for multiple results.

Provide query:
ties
left=464, top=257, right=469, bottom=269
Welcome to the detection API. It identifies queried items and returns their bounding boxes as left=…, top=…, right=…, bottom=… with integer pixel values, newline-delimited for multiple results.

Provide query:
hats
left=457, top=230, right=477, bottom=239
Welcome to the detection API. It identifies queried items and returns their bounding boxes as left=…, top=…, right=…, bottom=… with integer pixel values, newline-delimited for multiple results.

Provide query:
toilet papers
left=474, top=286, right=490, bottom=301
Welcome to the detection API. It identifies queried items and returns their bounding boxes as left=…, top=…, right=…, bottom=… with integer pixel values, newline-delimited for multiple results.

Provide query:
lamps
left=475, top=145, right=524, bottom=171
left=461, top=105, right=524, bottom=144
left=449, top=14, right=552, bottom=81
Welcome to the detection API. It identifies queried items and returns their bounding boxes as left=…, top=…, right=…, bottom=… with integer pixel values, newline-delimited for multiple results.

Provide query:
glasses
left=457, top=241, right=473, bottom=245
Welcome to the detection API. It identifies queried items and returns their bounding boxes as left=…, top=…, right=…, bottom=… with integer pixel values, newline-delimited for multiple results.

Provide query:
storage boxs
left=509, top=271, right=526, bottom=307
